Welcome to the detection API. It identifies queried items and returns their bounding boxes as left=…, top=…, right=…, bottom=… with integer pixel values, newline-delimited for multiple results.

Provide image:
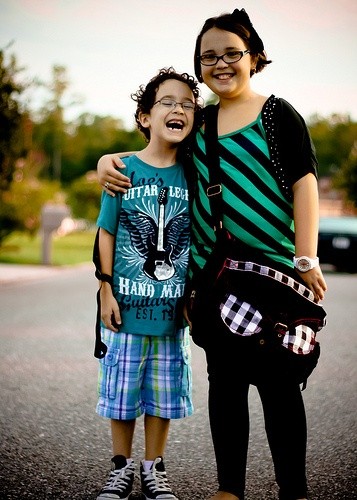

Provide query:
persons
left=92, top=67, right=199, bottom=499
left=96, top=8, right=328, bottom=500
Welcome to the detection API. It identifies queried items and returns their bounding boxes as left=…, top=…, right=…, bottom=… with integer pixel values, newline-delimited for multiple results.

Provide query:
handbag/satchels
left=185, top=240, right=327, bottom=391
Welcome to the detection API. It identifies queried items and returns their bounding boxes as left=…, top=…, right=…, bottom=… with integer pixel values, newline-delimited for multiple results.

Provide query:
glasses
left=153, top=99, right=196, bottom=113
left=197, top=49, right=251, bottom=66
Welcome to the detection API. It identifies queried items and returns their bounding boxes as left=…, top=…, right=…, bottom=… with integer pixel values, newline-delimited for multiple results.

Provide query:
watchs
left=292, top=255, right=320, bottom=273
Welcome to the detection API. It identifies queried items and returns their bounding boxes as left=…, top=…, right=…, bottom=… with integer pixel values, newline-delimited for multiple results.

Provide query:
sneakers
left=140, top=456, right=179, bottom=500
left=96, top=455, right=140, bottom=500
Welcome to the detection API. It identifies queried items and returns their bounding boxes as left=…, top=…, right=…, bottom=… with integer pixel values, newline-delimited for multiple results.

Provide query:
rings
left=104, top=181, right=110, bottom=189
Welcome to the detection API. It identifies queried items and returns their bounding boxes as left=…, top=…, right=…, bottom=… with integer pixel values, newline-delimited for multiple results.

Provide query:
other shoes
left=206, top=491, right=240, bottom=500
